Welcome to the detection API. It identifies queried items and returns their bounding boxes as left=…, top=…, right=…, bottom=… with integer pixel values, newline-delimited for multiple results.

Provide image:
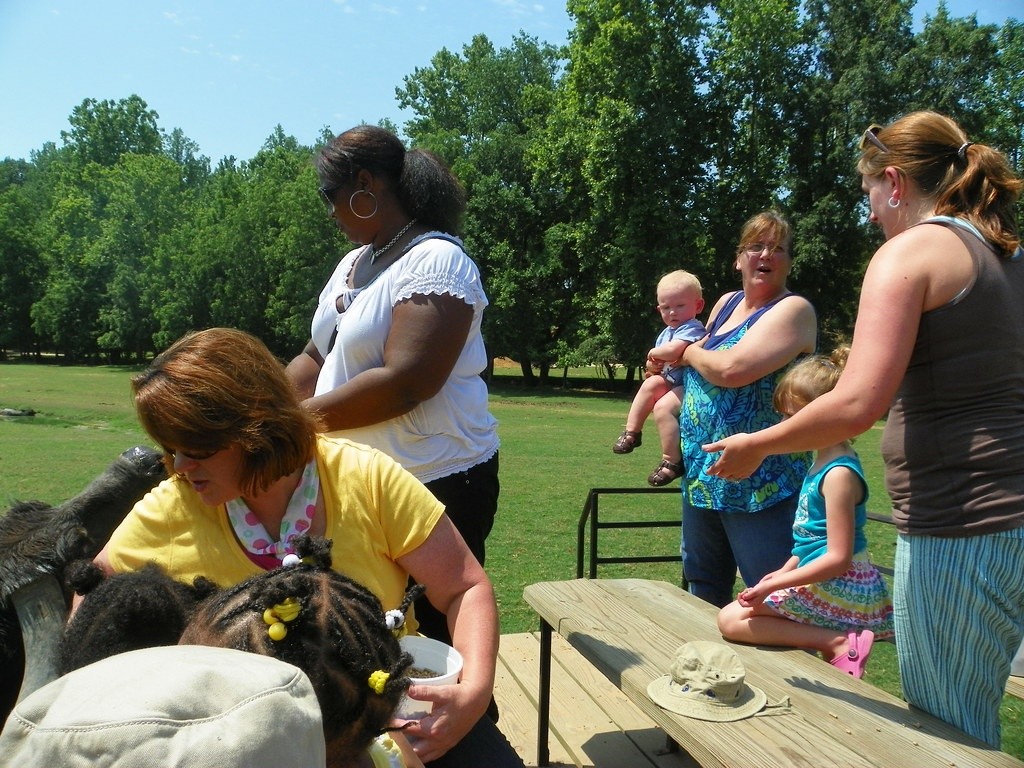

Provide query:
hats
left=0, top=645, right=327, bottom=768
left=647, top=641, right=791, bottom=723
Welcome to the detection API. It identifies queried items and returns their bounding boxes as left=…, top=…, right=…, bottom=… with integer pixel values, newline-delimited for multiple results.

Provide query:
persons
left=615, top=270, right=708, bottom=487
left=85, top=326, right=537, bottom=768
left=696, top=112, right=1024, bottom=752
left=279, top=124, right=503, bottom=725
left=171, top=533, right=425, bottom=768
left=669, top=212, right=820, bottom=608
left=51, top=558, right=226, bottom=680
left=717, top=339, right=898, bottom=681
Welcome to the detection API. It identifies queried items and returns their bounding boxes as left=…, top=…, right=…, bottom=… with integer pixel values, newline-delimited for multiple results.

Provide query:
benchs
left=476, top=577, right=1024, bottom=768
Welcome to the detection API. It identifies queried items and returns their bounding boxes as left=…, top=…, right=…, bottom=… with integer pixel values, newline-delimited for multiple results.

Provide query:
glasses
left=318, top=175, right=350, bottom=210
left=160, top=442, right=220, bottom=460
left=745, top=243, right=786, bottom=254
left=859, top=123, right=889, bottom=152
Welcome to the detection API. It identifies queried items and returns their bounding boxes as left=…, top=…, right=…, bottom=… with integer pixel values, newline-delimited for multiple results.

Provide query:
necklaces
left=369, top=216, right=419, bottom=267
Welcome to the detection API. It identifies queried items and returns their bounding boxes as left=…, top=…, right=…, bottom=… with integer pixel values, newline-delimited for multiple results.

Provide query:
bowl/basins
left=396, top=635, right=463, bottom=720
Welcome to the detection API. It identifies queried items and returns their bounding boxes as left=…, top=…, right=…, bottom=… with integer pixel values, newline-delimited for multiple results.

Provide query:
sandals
left=613, top=430, right=642, bottom=454
left=830, top=630, right=875, bottom=678
left=648, top=458, right=685, bottom=486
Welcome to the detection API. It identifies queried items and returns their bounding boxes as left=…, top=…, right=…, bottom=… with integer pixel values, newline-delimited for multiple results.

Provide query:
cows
left=0, top=445, right=171, bottom=734
